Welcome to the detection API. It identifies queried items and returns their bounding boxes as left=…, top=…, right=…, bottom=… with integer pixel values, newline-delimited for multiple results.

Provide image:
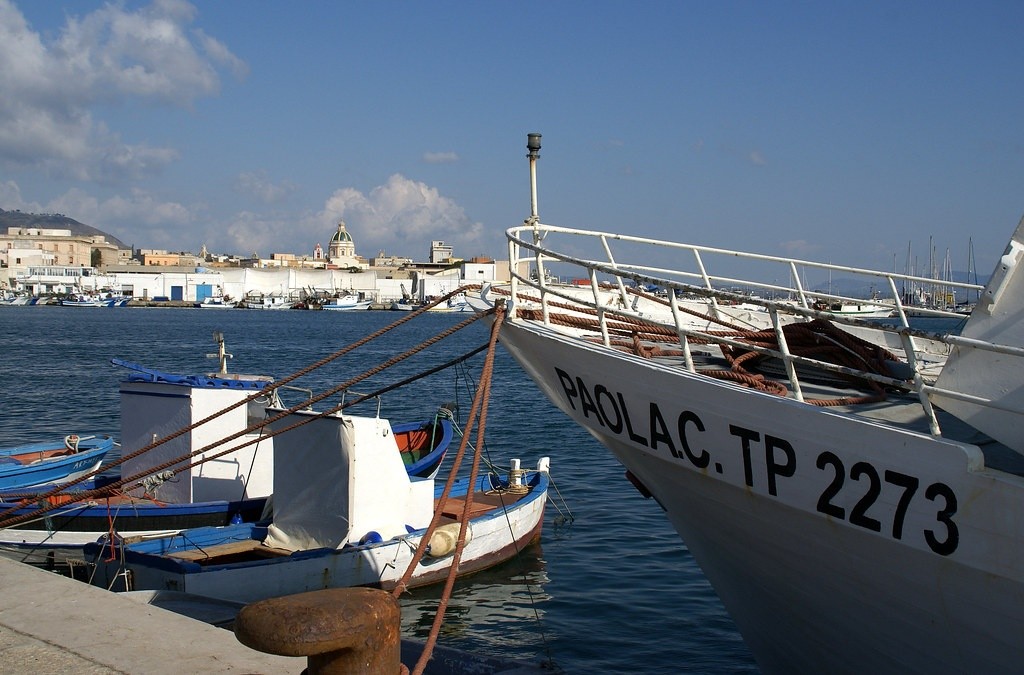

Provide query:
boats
left=0, top=433, right=122, bottom=494
left=463, top=134, right=1024, bottom=675
left=48, top=276, right=132, bottom=307
left=0, top=330, right=454, bottom=531
left=248, top=298, right=293, bottom=310
left=200, top=296, right=235, bottom=308
left=323, top=295, right=376, bottom=316
left=82, top=408, right=550, bottom=601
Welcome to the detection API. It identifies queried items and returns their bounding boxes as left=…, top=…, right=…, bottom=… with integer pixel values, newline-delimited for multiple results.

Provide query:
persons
left=447, top=298, right=452, bottom=309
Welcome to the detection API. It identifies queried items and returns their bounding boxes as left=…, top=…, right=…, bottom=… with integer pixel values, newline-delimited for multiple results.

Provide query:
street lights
left=154, top=274, right=165, bottom=296
